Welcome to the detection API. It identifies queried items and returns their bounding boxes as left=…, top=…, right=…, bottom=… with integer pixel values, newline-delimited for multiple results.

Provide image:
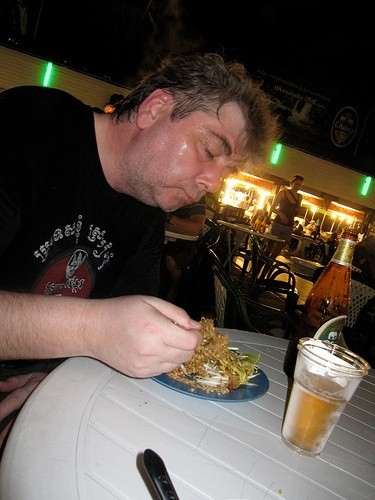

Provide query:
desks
left=216, top=219, right=284, bottom=285
left=281, top=233, right=316, bottom=258
left=164, top=230, right=199, bottom=296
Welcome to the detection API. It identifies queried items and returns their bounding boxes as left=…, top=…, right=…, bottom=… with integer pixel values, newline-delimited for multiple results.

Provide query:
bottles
left=283, top=228, right=358, bottom=373
left=252, top=215, right=267, bottom=234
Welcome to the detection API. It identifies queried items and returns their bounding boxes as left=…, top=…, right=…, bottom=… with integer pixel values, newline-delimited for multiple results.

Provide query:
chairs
left=248, top=234, right=300, bottom=316
left=170, top=219, right=221, bottom=303
left=209, top=250, right=316, bottom=342
left=303, top=235, right=334, bottom=266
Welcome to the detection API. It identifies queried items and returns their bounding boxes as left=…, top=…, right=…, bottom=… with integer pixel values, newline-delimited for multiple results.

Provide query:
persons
left=159, top=194, right=207, bottom=303
left=248, top=174, right=306, bottom=282
left=238, top=197, right=375, bottom=287
left=0, top=52, right=285, bottom=462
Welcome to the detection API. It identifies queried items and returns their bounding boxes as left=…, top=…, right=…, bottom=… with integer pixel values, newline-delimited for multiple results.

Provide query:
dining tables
left=1, top=328, right=375, bottom=500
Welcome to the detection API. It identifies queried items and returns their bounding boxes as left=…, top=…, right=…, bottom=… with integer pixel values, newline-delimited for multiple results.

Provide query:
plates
left=151, top=348, right=269, bottom=403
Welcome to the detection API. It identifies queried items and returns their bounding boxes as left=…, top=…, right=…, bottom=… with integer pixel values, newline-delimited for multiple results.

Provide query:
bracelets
left=166, top=212, right=174, bottom=226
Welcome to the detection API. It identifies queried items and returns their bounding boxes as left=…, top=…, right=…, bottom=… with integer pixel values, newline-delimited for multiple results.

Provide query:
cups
left=282, top=337, right=371, bottom=457
